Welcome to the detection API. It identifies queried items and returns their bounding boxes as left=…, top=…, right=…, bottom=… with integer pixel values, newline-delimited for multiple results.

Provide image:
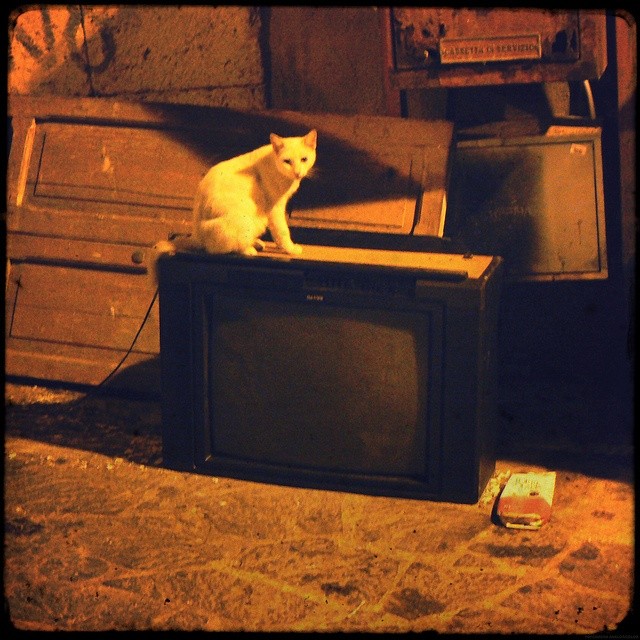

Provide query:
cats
left=146, top=129, right=318, bottom=285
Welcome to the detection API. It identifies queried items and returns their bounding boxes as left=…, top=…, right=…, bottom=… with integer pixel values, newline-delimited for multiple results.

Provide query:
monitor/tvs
left=153, top=231, right=504, bottom=505
